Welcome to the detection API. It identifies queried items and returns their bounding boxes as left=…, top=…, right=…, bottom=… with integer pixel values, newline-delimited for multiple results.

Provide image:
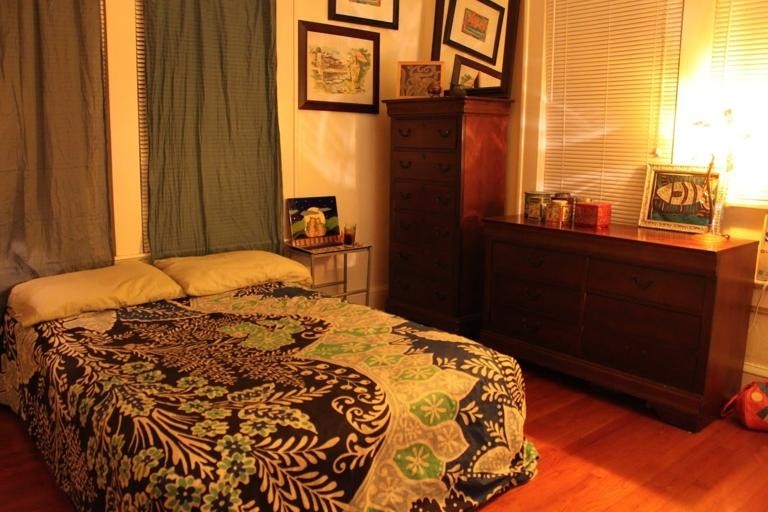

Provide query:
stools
left=286, top=241, right=373, bottom=309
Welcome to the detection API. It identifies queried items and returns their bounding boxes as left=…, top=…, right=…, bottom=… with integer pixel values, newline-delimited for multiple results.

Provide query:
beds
left=0, top=278, right=540, bottom=512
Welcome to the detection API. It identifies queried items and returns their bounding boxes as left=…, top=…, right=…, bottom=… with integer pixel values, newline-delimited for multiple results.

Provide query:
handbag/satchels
left=719, top=380, right=768, bottom=432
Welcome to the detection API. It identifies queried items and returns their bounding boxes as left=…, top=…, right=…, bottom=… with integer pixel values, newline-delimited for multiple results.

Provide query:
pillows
left=7, top=259, right=186, bottom=329
left=153, top=248, right=313, bottom=298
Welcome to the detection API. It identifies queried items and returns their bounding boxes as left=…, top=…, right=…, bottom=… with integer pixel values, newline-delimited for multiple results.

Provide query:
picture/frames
left=451, top=55, right=505, bottom=92
left=327, top=0, right=399, bottom=29
left=443, top=0, right=506, bottom=65
left=287, top=194, right=341, bottom=248
left=395, top=59, right=446, bottom=101
left=297, top=19, right=380, bottom=113
left=638, top=164, right=724, bottom=235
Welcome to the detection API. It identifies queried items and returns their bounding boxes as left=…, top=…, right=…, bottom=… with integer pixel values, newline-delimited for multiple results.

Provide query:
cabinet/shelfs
left=383, top=98, right=511, bottom=336
left=477, top=216, right=760, bottom=432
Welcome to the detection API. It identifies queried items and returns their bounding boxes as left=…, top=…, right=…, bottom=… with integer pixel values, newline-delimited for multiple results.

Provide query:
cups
left=344, top=225, right=355, bottom=247
